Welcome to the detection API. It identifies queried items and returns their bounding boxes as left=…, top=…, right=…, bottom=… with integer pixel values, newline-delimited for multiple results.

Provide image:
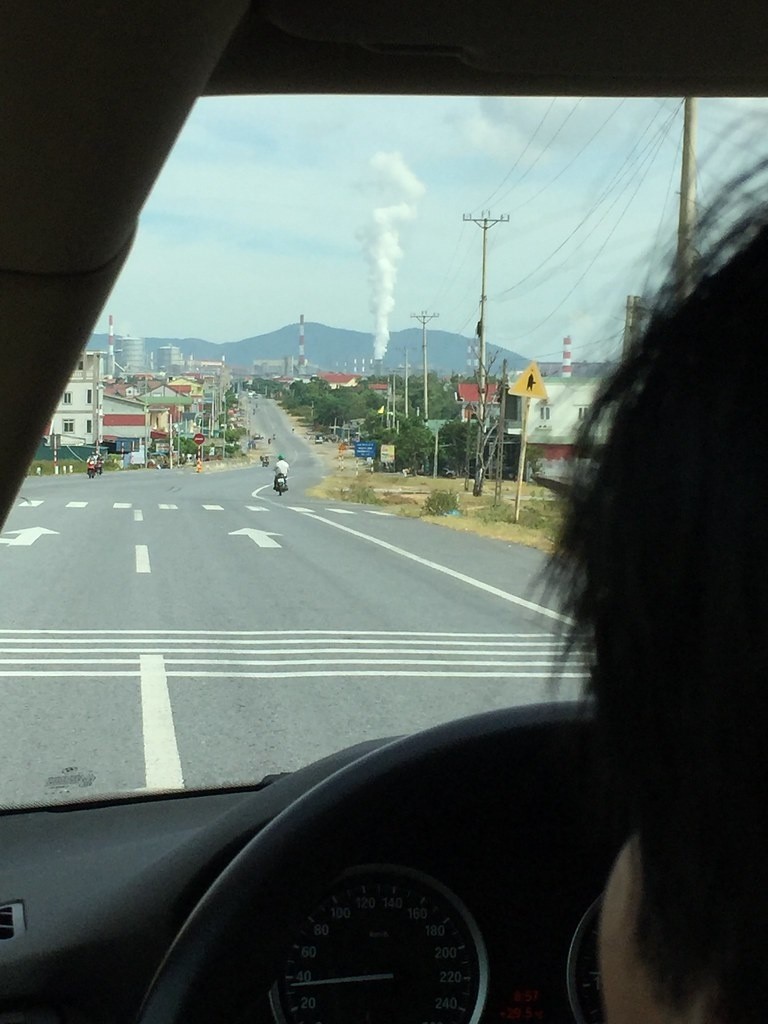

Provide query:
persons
left=85, top=451, right=103, bottom=477
left=272, top=453, right=290, bottom=489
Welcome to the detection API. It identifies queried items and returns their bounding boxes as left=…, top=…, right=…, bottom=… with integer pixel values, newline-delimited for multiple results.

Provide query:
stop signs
left=194, top=434, right=204, bottom=445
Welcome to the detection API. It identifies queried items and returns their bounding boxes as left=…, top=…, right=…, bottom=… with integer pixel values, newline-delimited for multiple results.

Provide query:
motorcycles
left=87, top=459, right=95, bottom=479
left=96, top=460, right=102, bottom=475
left=274, top=473, right=288, bottom=496
